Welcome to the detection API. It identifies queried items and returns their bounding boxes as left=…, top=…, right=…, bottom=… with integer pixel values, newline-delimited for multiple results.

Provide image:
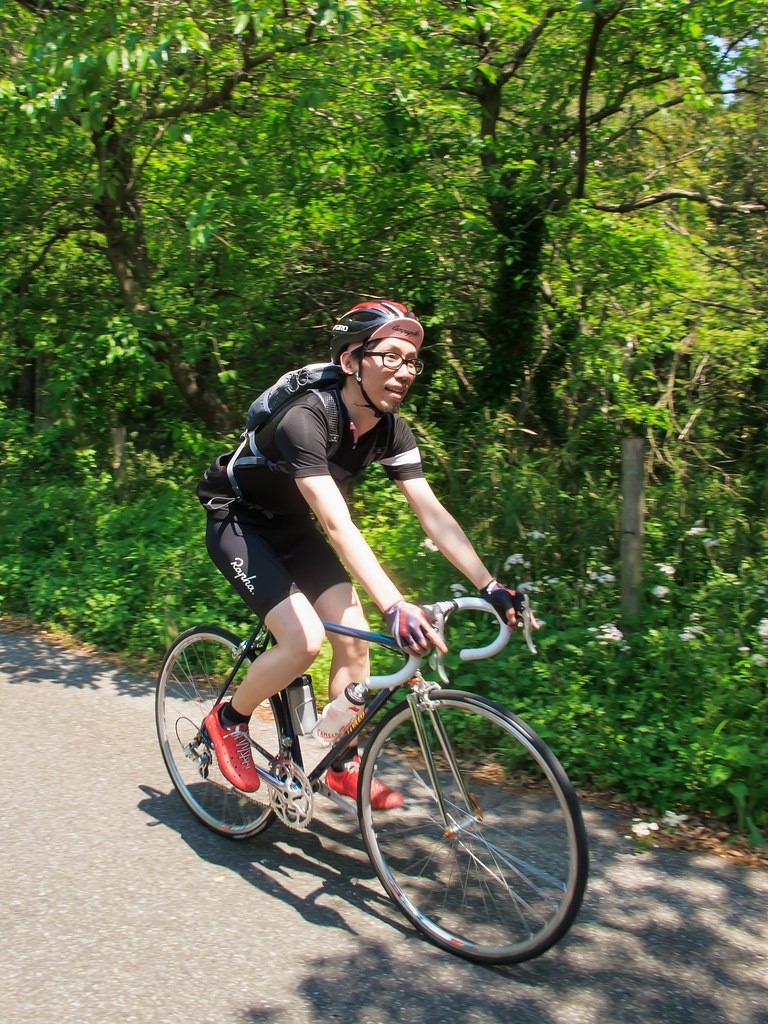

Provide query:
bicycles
left=153, top=585, right=591, bottom=964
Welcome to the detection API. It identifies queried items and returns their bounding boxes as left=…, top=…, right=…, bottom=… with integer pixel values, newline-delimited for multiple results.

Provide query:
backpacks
left=246, top=363, right=392, bottom=484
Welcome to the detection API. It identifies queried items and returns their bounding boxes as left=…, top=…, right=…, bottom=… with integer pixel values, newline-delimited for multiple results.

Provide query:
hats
left=347, top=318, right=424, bottom=352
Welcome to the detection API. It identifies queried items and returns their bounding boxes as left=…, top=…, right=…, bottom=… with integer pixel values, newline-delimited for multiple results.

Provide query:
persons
left=202, top=299, right=542, bottom=812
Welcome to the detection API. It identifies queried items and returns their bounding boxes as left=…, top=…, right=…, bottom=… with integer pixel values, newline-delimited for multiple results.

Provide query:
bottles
left=310, top=681, right=370, bottom=747
left=286, top=674, right=318, bottom=736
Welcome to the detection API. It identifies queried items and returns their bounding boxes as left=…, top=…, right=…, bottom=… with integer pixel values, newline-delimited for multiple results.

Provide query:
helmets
left=330, top=299, right=423, bottom=364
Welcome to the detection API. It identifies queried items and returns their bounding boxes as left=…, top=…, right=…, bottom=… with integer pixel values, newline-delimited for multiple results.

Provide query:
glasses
left=362, top=352, right=426, bottom=375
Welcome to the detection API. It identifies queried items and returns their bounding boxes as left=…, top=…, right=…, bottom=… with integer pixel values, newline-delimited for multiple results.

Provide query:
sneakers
left=199, top=703, right=261, bottom=793
left=324, top=756, right=404, bottom=810
